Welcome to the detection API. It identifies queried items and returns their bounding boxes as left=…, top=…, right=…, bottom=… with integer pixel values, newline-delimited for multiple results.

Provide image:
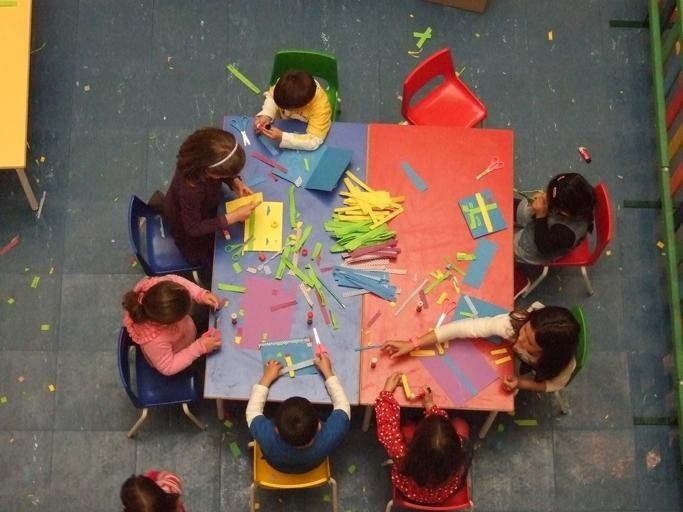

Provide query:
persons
left=244, top=352, right=351, bottom=476
left=254, top=69, right=332, bottom=152
left=514, top=172, right=596, bottom=295
left=122, top=274, right=222, bottom=377
left=380, top=306, right=579, bottom=395
left=120, top=469, right=185, bottom=512
left=375, top=373, right=470, bottom=505
left=162, top=126, right=255, bottom=268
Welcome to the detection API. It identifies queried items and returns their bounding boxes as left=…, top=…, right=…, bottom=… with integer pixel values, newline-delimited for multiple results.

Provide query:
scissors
left=228, top=115, right=252, bottom=147
left=476, top=156, right=505, bottom=183
left=223, top=234, right=256, bottom=262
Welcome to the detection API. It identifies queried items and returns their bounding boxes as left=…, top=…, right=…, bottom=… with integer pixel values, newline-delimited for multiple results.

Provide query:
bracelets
left=409, top=334, right=420, bottom=351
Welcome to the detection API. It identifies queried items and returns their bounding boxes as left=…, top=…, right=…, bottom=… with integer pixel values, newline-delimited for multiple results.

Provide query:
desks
left=204, top=114, right=361, bottom=423
left=362, top=122, right=514, bottom=438
left=1, top=1, right=40, bottom=212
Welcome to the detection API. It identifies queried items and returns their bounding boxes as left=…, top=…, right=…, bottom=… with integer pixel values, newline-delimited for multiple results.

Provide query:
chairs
left=513, top=303, right=589, bottom=423
left=513, top=182, right=614, bottom=299
left=248, top=431, right=341, bottom=510
left=400, top=51, right=488, bottom=127
left=272, top=48, right=343, bottom=122
left=116, top=326, right=203, bottom=436
left=383, top=419, right=477, bottom=511
left=128, top=191, right=207, bottom=281
